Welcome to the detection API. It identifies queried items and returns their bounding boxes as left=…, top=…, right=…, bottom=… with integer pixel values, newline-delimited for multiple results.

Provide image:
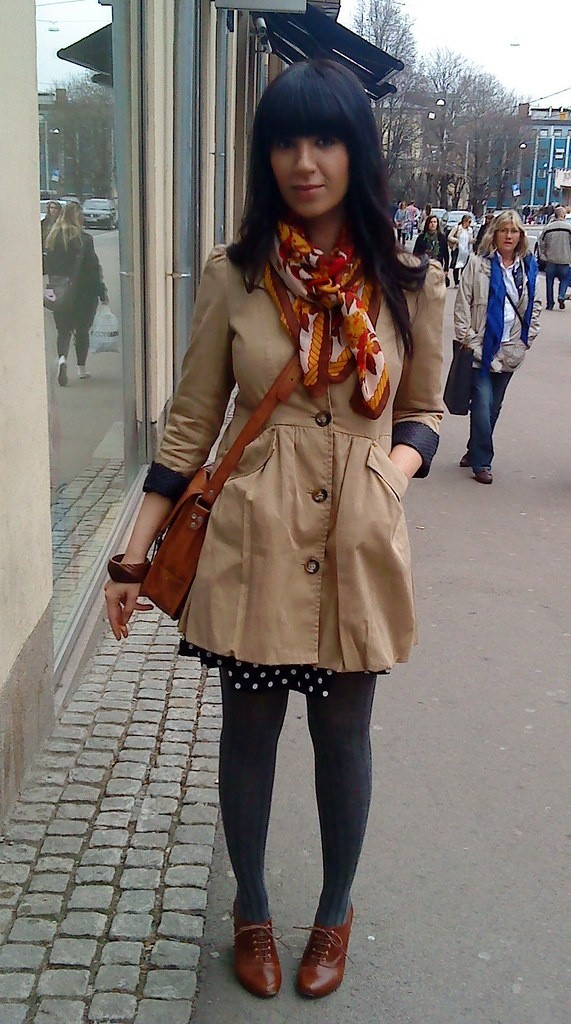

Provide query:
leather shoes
left=299, top=892, right=352, bottom=997
left=460, top=454, right=469, bottom=466
left=475, top=469, right=493, bottom=483
left=232, top=894, right=291, bottom=996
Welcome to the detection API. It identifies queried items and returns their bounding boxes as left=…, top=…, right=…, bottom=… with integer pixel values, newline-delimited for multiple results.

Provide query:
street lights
left=45, top=121, right=60, bottom=190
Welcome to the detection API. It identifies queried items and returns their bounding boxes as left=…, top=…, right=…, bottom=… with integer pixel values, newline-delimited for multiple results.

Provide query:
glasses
left=469, top=220, right=471, bottom=223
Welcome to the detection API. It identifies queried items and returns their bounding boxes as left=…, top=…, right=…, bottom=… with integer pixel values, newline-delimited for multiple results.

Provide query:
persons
left=539, top=207, right=571, bottom=311
left=40, top=201, right=110, bottom=386
left=454, top=210, right=542, bottom=484
left=103, top=61, right=445, bottom=999
left=473, top=213, right=494, bottom=255
left=522, top=202, right=571, bottom=224
left=392, top=199, right=432, bottom=246
left=448, top=215, right=477, bottom=288
left=413, top=215, right=450, bottom=279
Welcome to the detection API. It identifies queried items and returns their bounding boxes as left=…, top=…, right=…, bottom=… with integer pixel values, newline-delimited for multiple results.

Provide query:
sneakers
left=546, top=307, right=552, bottom=310
left=559, top=298, right=565, bottom=309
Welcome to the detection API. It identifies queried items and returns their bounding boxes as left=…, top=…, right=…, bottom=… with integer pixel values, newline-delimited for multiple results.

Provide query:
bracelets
left=108, top=554, right=150, bottom=583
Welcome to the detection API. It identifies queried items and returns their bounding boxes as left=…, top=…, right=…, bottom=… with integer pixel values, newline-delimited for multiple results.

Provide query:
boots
left=57, top=356, right=67, bottom=386
left=78, top=365, right=91, bottom=378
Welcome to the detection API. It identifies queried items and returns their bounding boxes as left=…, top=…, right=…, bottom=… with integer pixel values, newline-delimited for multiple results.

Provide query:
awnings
left=271, top=16, right=397, bottom=102
left=269, top=34, right=371, bottom=104
left=285, top=3, right=404, bottom=86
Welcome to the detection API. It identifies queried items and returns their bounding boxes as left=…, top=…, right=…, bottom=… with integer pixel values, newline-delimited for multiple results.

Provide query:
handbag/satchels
left=139, top=468, right=213, bottom=620
left=443, top=339, right=474, bottom=416
left=449, top=225, right=459, bottom=249
left=89, top=305, right=121, bottom=353
left=43, top=275, right=77, bottom=316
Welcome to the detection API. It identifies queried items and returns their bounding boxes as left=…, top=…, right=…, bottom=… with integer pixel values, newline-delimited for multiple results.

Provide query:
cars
left=522, top=213, right=571, bottom=272
left=414, top=208, right=478, bottom=237
left=82, top=193, right=119, bottom=229
left=41, top=192, right=80, bottom=220
